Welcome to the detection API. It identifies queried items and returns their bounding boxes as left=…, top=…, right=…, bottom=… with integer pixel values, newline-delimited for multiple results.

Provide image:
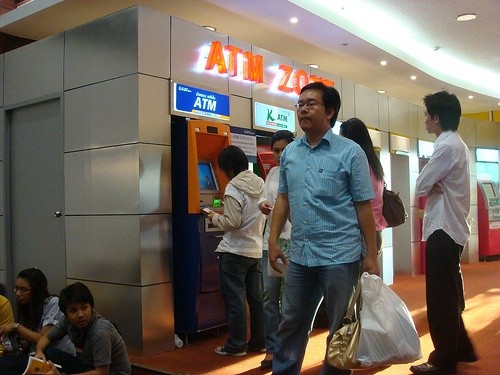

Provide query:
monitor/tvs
left=198, top=161, right=219, bottom=190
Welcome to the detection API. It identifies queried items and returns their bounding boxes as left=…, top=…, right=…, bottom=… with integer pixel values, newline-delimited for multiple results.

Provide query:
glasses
left=13, top=287, right=31, bottom=293
left=294, top=103, right=317, bottom=109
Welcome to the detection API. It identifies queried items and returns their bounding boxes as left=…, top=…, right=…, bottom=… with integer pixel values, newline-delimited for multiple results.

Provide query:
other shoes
left=261, top=360, right=272, bottom=367
left=215, top=346, right=247, bottom=356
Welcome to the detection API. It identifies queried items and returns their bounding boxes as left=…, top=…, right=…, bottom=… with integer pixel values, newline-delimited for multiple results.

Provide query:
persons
left=0, top=268, right=76, bottom=357
left=268, top=81, right=380, bottom=375
left=34, top=282, right=132, bottom=375
left=0, top=282, right=15, bottom=355
left=207, top=145, right=266, bottom=357
left=409, top=91, right=478, bottom=375
left=339, top=118, right=388, bottom=321
left=261, top=130, right=296, bottom=368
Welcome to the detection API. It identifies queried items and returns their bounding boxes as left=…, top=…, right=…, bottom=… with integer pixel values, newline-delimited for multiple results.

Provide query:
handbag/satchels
left=325, top=281, right=369, bottom=370
left=382, top=181, right=408, bottom=228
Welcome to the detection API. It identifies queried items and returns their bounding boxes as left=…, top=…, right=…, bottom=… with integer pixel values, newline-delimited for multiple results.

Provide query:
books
left=21, top=356, right=62, bottom=375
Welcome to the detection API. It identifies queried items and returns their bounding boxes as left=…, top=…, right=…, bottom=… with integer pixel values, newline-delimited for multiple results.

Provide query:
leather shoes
left=410, top=361, right=455, bottom=375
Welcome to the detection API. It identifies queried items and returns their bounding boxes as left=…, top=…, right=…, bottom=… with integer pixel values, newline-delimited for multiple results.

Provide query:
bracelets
left=14, top=323, right=20, bottom=331
